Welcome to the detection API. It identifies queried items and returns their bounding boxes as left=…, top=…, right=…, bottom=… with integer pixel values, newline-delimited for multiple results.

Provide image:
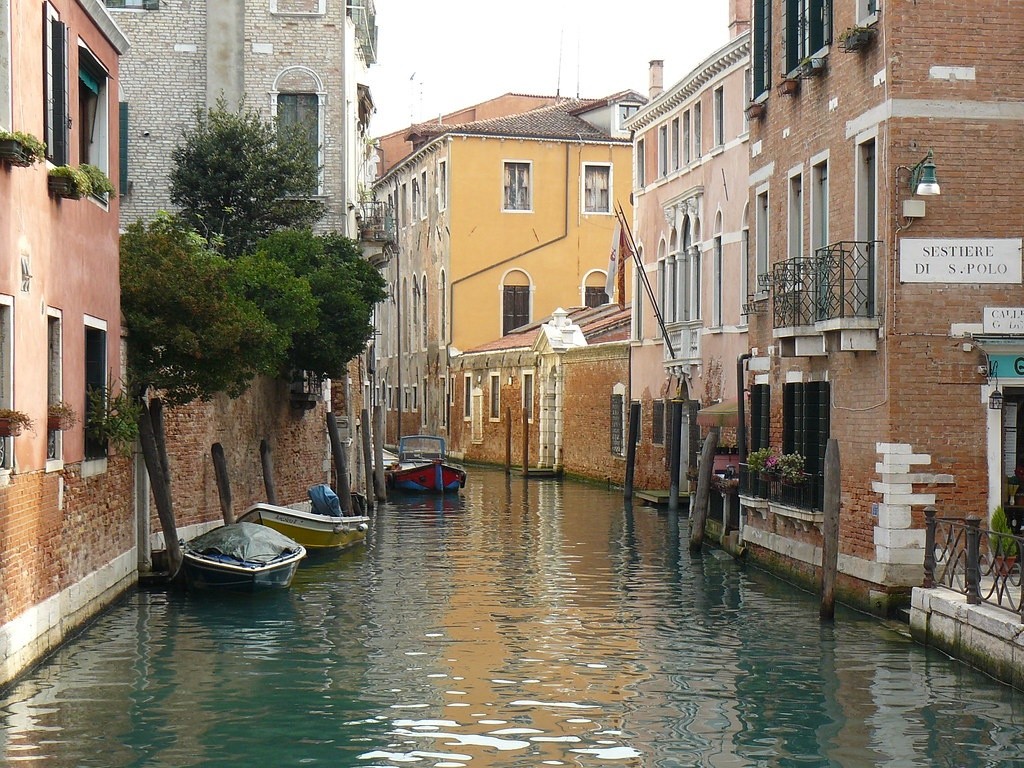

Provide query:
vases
left=760, top=472, right=775, bottom=481
left=781, top=475, right=794, bottom=486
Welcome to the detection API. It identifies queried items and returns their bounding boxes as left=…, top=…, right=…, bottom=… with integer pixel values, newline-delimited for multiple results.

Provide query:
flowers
left=744, top=447, right=779, bottom=471
left=781, top=453, right=808, bottom=482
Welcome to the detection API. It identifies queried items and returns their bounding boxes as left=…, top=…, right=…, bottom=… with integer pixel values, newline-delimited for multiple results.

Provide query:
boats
left=179, top=521, right=307, bottom=592
left=370, top=434, right=466, bottom=495
left=236, top=491, right=370, bottom=552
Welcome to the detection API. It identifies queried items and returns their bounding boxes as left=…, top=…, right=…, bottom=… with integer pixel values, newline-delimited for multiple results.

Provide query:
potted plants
left=0, top=129, right=47, bottom=169
left=48, top=163, right=117, bottom=208
left=779, top=77, right=798, bottom=94
left=0, top=407, right=36, bottom=437
left=686, top=467, right=738, bottom=495
left=359, top=218, right=374, bottom=241
left=47, top=400, right=80, bottom=431
left=837, top=25, right=869, bottom=50
left=85, top=368, right=142, bottom=462
left=799, top=57, right=823, bottom=76
left=745, top=103, right=762, bottom=120
left=989, top=506, right=1018, bottom=577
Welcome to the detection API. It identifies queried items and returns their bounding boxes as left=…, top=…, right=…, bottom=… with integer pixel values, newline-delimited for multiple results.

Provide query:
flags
left=605, top=220, right=634, bottom=312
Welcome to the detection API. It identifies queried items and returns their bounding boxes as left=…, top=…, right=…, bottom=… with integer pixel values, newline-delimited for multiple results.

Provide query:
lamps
left=987, top=356, right=1004, bottom=410
left=910, top=148, right=941, bottom=197
